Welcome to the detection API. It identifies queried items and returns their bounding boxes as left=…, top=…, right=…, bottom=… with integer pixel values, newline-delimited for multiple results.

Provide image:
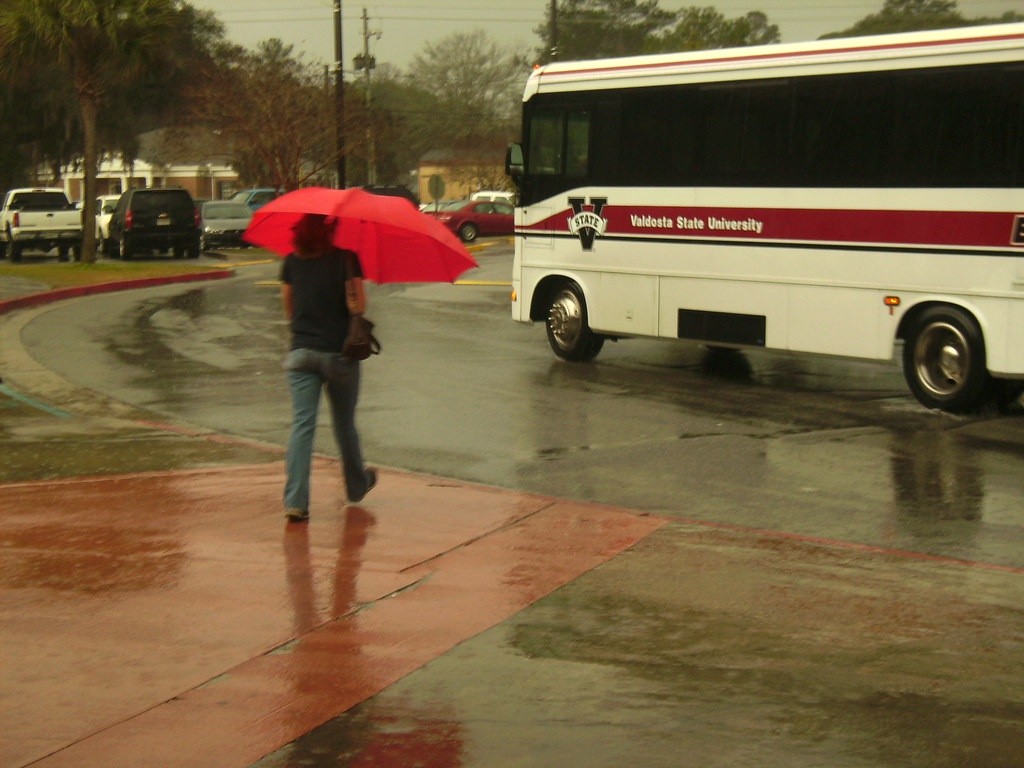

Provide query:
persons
left=278, top=213, right=378, bottom=523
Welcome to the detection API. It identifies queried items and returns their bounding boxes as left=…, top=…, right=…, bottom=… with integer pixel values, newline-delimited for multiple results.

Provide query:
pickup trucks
left=0, top=187, right=84, bottom=263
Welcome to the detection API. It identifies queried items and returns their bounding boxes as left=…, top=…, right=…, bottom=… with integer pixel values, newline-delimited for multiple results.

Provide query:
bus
left=505, top=22, right=1024, bottom=413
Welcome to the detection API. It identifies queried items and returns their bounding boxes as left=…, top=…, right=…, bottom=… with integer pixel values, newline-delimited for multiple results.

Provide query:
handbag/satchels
left=335, top=315, right=381, bottom=368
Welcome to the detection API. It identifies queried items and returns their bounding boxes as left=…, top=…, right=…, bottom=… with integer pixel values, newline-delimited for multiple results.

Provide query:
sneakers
left=348, top=467, right=377, bottom=502
left=284, top=508, right=311, bottom=519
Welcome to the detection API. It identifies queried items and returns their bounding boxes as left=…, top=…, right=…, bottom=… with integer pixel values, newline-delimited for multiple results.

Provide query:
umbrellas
left=242, top=187, right=480, bottom=286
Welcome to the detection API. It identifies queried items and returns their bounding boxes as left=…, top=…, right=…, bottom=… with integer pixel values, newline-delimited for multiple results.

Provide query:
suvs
left=102, top=185, right=202, bottom=260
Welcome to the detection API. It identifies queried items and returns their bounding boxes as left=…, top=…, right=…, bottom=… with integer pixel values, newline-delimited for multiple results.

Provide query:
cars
left=76, top=185, right=515, bottom=252
